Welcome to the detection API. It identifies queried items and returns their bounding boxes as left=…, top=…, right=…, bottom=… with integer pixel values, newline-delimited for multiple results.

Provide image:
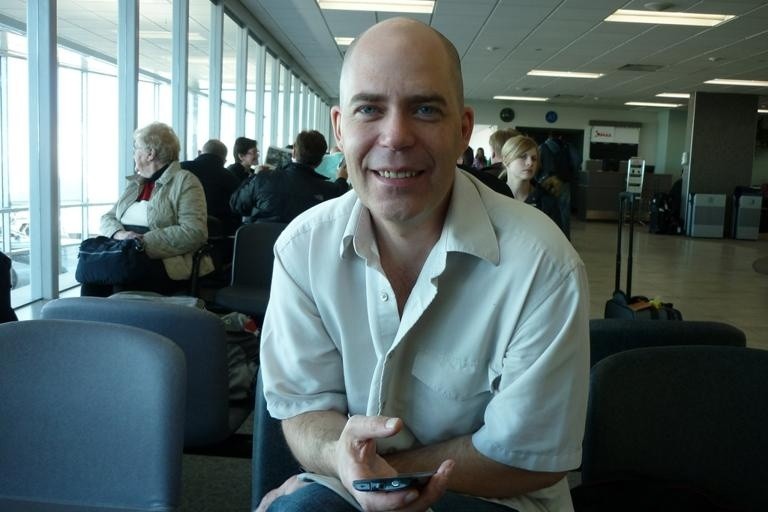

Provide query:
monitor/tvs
left=620, top=161, right=628, bottom=172
left=645, top=165, right=654, bottom=173
left=586, top=160, right=603, bottom=172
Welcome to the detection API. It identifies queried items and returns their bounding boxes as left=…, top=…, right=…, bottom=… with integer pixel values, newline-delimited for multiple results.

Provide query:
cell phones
left=353, top=472, right=433, bottom=492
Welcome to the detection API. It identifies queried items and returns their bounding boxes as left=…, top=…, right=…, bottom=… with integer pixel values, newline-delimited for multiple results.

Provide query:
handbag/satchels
left=75, top=236, right=151, bottom=286
left=106, top=290, right=260, bottom=410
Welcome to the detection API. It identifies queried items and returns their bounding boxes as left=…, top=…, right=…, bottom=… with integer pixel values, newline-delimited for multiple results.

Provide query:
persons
left=82, top=122, right=213, bottom=296
left=0, top=249, right=21, bottom=327
left=177, top=130, right=352, bottom=239
left=259, top=17, right=591, bottom=510
left=461, top=128, right=570, bottom=237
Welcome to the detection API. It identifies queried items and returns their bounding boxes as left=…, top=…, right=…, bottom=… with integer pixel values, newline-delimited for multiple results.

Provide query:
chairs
left=0, top=216, right=768, bottom=511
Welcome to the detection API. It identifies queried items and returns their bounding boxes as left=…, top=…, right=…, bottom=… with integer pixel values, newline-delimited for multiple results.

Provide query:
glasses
left=132, top=145, right=149, bottom=152
left=247, top=151, right=260, bottom=158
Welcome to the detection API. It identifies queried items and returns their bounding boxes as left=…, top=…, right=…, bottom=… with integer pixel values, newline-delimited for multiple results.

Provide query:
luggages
left=606, top=192, right=684, bottom=321
left=648, top=192, right=673, bottom=234
